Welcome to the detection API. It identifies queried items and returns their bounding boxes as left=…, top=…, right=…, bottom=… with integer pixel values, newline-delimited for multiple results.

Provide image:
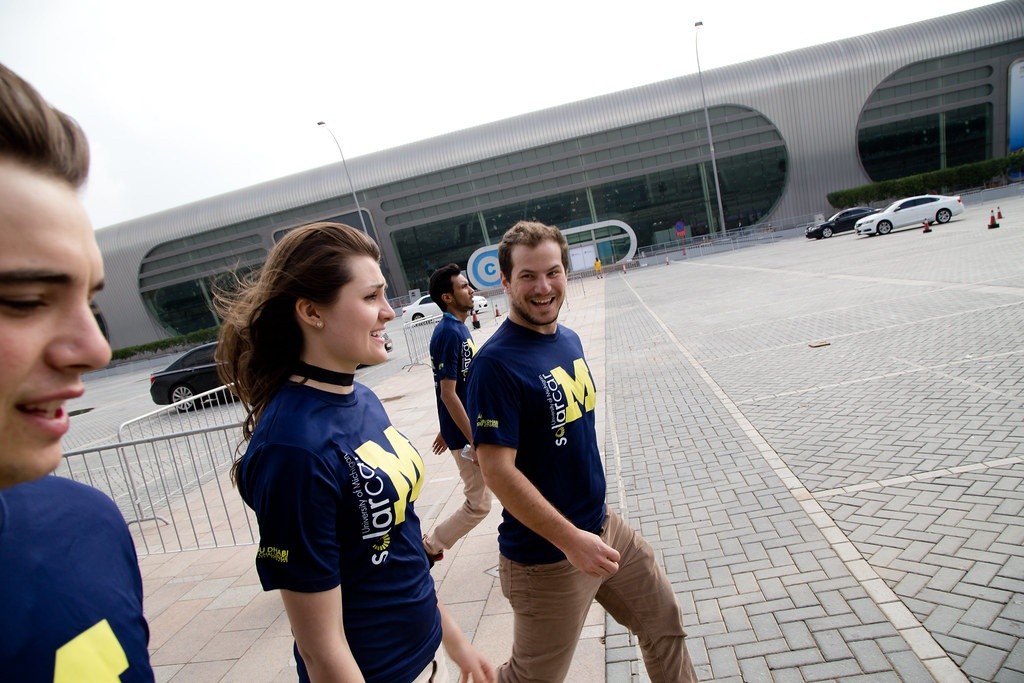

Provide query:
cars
left=804, top=206, right=883, bottom=240
left=854, top=193, right=965, bottom=237
left=402, top=293, right=489, bottom=327
left=149, top=341, right=239, bottom=413
left=355, top=332, right=394, bottom=370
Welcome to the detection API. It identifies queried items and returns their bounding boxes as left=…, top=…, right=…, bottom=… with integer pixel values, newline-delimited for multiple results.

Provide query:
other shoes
left=424, top=546, right=443, bottom=570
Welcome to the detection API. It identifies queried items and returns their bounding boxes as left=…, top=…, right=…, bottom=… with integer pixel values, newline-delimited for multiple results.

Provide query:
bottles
left=460, top=444, right=475, bottom=461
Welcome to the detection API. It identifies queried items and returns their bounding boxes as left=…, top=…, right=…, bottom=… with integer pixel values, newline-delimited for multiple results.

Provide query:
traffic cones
left=495, top=303, right=502, bottom=317
left=473, top=308, right=480, bottom=328
left=997, top=206, right=1003, bottom=219
left=922, top=217, right=932, bottom=233
left=987, top=209, right=1000, bottom=229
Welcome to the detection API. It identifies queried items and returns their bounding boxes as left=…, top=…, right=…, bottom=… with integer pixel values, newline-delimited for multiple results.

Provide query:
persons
left=214, top=221, right=498, bottom=683
left=0, top=64, right=154, bottom=683
left=465, top=221, right=698, bottom=683
left=421, top=264, right=480, bottom=573
left=594, top=257, right=603, bottom=279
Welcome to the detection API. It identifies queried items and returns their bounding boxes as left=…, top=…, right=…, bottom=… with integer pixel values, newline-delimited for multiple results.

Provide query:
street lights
left=693, top=21, right=728, bottom=239
left=315, top=122, right=368, bottom=233
